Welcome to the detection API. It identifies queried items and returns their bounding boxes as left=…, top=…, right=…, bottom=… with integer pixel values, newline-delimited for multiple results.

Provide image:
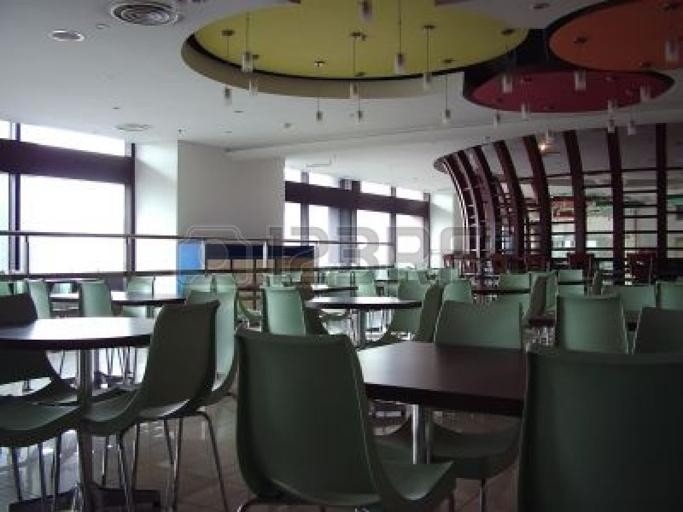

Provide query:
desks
left=355, top=339, right=526, bottom=465
left=0, top=317, right=160, bottom=512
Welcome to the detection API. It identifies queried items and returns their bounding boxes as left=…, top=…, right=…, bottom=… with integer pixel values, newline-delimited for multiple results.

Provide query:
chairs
left=376, top=300, right=524, bottom=512
left=518, top=343, right=683, bottom=512
left=80, top=299, right=221, bottom=512
left=130, top=290, right=239, bottom=512
left=0, top=396, right=81, bottom=512
left=233, top=327, right=457, bottom=512
left=0, top=293, right=135, bottom=512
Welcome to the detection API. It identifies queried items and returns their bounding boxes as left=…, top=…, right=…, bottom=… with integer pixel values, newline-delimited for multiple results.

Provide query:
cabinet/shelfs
left=176, top=239, right=315, bottom=310
